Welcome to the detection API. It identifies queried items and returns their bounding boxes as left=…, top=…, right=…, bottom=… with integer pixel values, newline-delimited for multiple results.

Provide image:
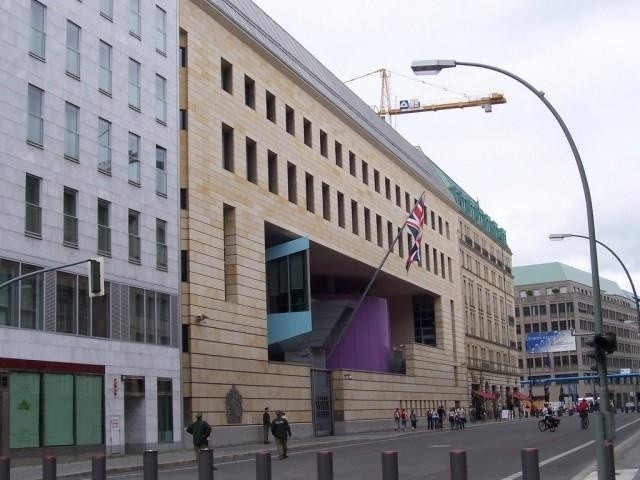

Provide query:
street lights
left=407, top=58, right=621, bottom=480
left=545, top=233, right=640, bottom=335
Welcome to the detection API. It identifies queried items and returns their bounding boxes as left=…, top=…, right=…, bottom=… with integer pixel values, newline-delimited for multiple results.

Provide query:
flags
left=404, top=196, right=427, bottom=275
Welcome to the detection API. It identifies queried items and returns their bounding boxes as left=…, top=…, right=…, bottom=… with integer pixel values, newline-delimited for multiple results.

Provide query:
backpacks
left=545, top=407, right=553, bottom=415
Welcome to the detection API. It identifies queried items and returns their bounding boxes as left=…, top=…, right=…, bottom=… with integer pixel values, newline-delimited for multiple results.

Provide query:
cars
left=625, top=401, right=634, bottom=410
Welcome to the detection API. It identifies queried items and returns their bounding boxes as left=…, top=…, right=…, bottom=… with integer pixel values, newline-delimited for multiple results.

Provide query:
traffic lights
left=585, top=339, right=598, bottom=373
left=596, top=331, right=620, bottom=354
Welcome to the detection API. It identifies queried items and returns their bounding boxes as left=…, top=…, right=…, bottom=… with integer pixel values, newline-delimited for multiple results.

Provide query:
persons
left=270, top=409, right=292, bottom=460
left=426, top=404, right=486, bottom=431
left=409, top=409, right=417, bottom=431
left=400, top=408, right=408, bottom=432
left=541, top=402, right=556, bottom=429
left=281, top=412, right=288, bottom=444
left=578, top=398, right=589, bottom=431
left=393, top=407, right=401, bottom=432
left=262, top=406, right=272, bottom=445
left=493, top=399, right=601, bottom=424
left=185, top=412, right=218, bottom=471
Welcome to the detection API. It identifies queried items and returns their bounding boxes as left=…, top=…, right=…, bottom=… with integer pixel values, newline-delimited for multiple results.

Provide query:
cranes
left=341, top=66, right=509, bottom=121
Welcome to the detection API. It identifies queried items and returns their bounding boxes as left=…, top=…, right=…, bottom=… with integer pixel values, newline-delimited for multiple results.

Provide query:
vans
left=577, top=396, right=600, bottom=406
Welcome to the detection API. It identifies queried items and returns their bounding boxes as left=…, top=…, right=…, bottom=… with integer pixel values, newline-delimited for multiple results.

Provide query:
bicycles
left=536, top=416, right=556, bottom=433
left=582, top=410, right=590, bottom=432
